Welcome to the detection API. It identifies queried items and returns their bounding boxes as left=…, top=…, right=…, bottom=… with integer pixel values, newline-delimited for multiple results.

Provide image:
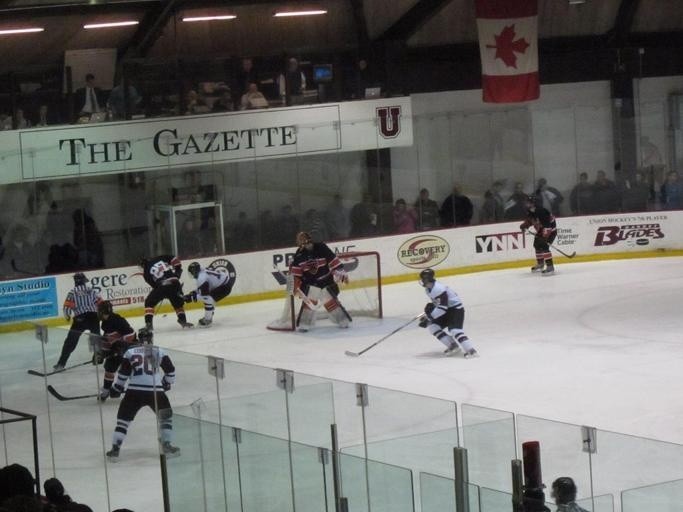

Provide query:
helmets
left=296, top=232, right=312, bottom=246
left=138, top=328, right=152, bottom=341
left=420, top=269, right=434, bottom=286
left=97, top=301, right=112, bottom=319
left=552, top=477, right=575, bottom=504
left=188, top=262, right=200, bottom=278
left=74, top=273, right=89, bottom=286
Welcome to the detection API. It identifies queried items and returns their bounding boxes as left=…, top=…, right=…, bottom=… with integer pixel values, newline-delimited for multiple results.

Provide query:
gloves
left=92, top=354, right=103, bottom=365
left=185, top=294, right=197, bottom=303
left=110, top=389, right=120, bottom=397
left=162, top=379, right=172, bottom=390
left=419, top=317, right=430, bottom=328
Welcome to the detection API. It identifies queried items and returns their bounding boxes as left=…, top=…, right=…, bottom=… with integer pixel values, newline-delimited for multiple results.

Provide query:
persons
left=137, top=254, right=192, bottom=331
left=177, top=184, right=474, bottom=258
left=418, top=267, right=479, bottom=359
left=550, top=475, right=589, bottom=512
left=292, top=232, right=349, bottom=327
left=52, top=273, right=104, bottom=365
left=93, top=301, right=136, bottom=400
left=481, top=177, right=564, bottom=225
left=180, top=262, right=231, bottom=324
left=105, top=328, right=181, bottom=456
left=519, top=195, right=559, bottom=273
left=2, top=183, right=104, bottom=280
left=0, top=463, right=131, bottom=512
left=1, top=51, right=385, bottom=129
left=570, top=169, right=682, bottom=216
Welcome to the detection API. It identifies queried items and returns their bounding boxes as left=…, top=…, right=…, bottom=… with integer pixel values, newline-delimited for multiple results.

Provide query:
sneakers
left=198, top=319, right=211, bottom=326
left=532, top=264, right=543, bottom=270
left=162, top=446, right=180, bottom=453
left=542, top=267, right=553, bottom=273
left=98, top=391, right=109, bottom=399
left=444, top=344, right=457, bottom=352
left=107, top=450, right=118, bottom=456
left=464, top=348, right=475, bottom=356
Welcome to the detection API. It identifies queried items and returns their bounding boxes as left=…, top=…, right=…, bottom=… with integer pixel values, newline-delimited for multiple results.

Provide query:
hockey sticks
left=47, top=384, right=127, bottom=400
left=28, top=354, right=112, bottom=377
left=527, top=229, right=576, bottom=259
left=273, top=264, right=322, bottom=311
left=344, top=312, right=426, bottom=357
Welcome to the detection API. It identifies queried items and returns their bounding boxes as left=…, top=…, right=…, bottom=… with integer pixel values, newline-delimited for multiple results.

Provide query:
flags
left=471, top=0, right=540, bottom=103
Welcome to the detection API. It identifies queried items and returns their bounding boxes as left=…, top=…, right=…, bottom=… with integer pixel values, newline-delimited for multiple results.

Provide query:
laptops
left=365, top=87, right=381, bottom=98
left=89, top=112, right=107, bottom=122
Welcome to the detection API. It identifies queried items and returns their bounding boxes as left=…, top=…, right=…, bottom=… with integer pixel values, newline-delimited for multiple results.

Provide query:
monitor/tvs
left=312, top=64, right=333, bottom=82
